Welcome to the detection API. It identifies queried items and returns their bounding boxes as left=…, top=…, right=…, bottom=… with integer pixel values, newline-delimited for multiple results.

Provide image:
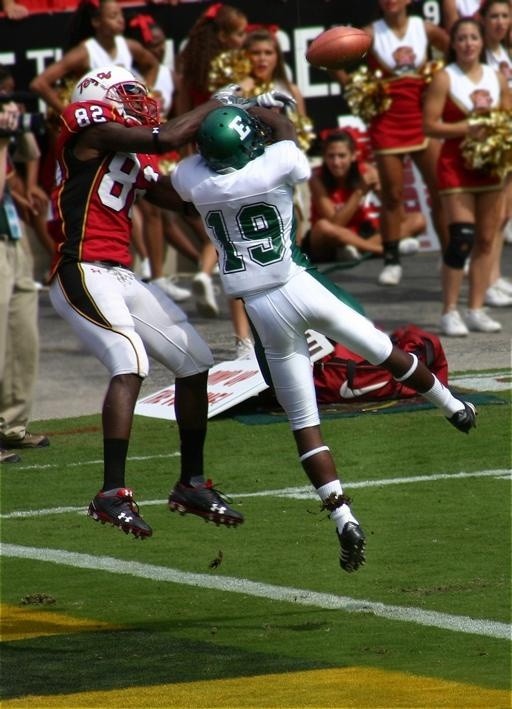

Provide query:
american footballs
left=307, top=25, right=373, bottom=70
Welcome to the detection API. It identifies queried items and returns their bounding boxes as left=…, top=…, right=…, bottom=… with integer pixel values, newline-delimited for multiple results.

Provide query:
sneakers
left=88, top=487, right=152, bottom=540
left=140, top=256, right=254, bottom=360
left=445, top=399, right=477, bottom=433
left=339, top=240, right=417, bottom=286
left=442, top=277, right=512, bottom=336
left=336, top=522, right=366, bottom=573
left=169, top=482, right=242, bottom=528
left=0, top=430, right=49, bottom=463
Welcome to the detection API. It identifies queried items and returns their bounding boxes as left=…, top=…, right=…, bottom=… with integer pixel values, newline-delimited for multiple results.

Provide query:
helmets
left=72, top=66, right=146, bottom=114
left=197, top=106, right=264, bottom=172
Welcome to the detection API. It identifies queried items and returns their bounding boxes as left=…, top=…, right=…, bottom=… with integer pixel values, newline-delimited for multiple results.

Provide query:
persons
left=39, top=65, right=298, bottom=542
left=168, top=104, right=478, bottom=575
left=1, top=1, right=512, bottom=465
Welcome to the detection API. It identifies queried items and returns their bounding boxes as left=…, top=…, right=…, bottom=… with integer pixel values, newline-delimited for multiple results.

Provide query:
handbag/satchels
left=313, top=325, right=448, bottom=403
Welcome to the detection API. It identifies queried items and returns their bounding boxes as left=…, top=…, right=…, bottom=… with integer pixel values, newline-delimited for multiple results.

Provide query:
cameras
left=0, top=111, right=45, bottom=135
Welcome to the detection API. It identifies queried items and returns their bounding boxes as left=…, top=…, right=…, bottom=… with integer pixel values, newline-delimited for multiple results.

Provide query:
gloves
left=211, top=83, right=297, bottom=111
